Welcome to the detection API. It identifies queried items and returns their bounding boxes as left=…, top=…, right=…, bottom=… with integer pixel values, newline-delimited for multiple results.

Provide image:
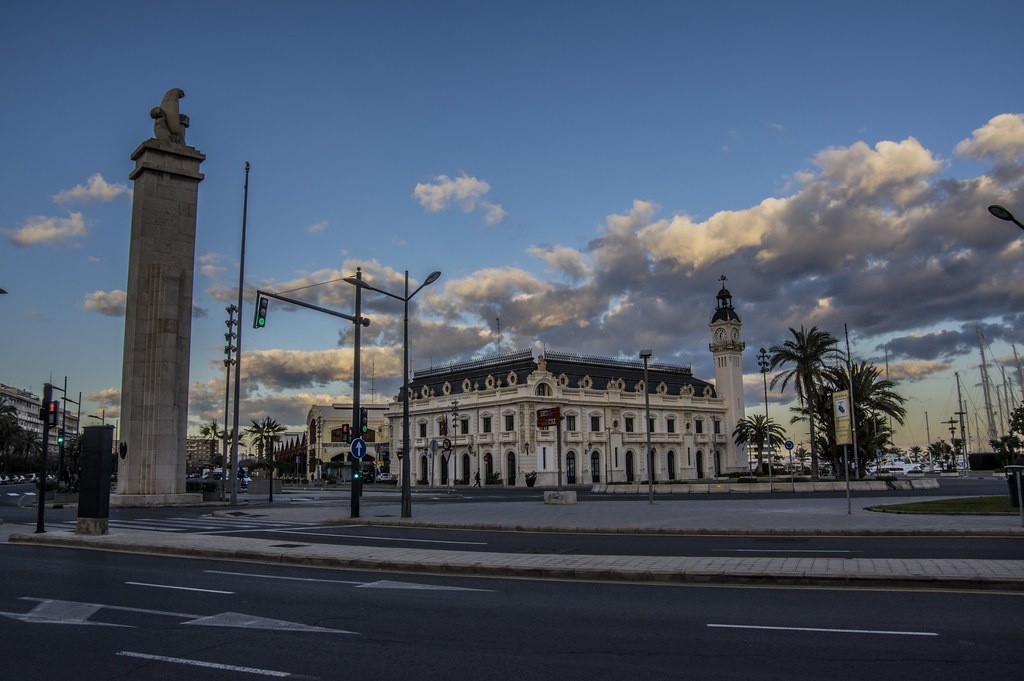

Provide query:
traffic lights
left=360, top=407, right=368, bottom=433
left=58, top=430, right=63, bottom=443
left=258, top=297, right=268, bottom=328
left=47, top=400, right=59, bottom=429
left=342, top=424, right=349, bottom=441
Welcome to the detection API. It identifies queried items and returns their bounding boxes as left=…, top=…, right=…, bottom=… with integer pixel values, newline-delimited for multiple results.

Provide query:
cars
left=0, top=473, right=56, bottom=484
left=184, top=467, right=252, bottom=487
left=376, top=472, right=390, bottom=482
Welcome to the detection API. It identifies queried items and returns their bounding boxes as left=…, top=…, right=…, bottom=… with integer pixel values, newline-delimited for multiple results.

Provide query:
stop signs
left=397, top=452, right=404, bottom=458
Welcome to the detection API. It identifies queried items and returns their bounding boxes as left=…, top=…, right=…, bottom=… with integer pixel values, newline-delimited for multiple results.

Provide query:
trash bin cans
left=1004, top=465, right=1024, bottom=508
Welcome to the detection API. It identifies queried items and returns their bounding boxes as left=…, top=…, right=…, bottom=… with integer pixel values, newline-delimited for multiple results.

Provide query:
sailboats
left=748, top=325, right=971, bottom=472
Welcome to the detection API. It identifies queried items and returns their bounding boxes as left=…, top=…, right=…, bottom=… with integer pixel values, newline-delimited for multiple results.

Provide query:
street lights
left=752, top=349, right=775, bottom=495
left=60, top=396, right=80, bottom=435
left=87, top=414, right=104, bottom=425
left=343, top=270, right=442, bottom=518
left=221, top=303, right=239, bottom=500
left=53, top=386, right=66, bottom=434
left=106, top=422, right=118, bottom=482
left=638, top=349, right=656, bottom=506
left=450, top=399, right=460, bottom=486
left=268, top=434, right=275, bottom=504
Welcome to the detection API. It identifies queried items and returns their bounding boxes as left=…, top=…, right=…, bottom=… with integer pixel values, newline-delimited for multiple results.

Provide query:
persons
left=473, top=472, right=481, bottom=488
left=238, top=467, right=245, bottom=481
left=311, top=474, right=314, bottom=482
